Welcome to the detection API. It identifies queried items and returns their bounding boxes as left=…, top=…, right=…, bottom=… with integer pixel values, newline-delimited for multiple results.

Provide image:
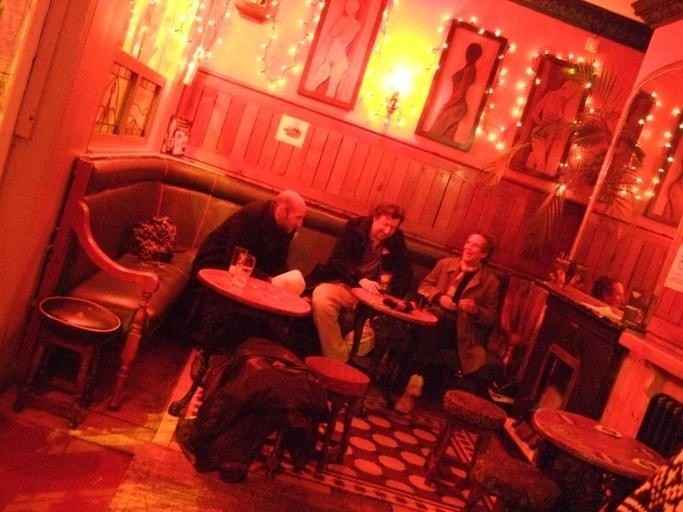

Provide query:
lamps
left=173, top=0, right=326, bottom=92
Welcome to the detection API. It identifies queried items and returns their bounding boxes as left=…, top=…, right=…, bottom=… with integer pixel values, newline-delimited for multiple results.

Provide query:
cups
left=377, top=269, right=393, bottom=298
left=227, top=246, right=255, bottom=290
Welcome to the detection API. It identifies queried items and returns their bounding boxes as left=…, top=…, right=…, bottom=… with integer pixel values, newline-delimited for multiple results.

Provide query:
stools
left=303, top=356, right=370, bottom=464
left=422, top=390, right=506, bottom=491
left=463, top=452, right=562, bottom=512
left=14, top=324, right=101, bottom=430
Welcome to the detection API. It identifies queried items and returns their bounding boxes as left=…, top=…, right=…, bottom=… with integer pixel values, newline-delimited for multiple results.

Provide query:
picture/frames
left=297, top=0, right=388, bottom=112
left=415, top=19, right=508, bottom=152
left=506, top=54, right=595, bottom=183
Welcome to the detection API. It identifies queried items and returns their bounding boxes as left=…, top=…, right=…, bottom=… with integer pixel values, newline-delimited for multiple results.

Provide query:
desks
left=347, top=288, right=439, bottom=417
left=169, top=268, right=311, bottom=418
left=531, top=408, right=667, bottom=512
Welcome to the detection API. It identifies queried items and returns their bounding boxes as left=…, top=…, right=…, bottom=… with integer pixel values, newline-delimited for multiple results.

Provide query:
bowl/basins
left=39, top=296, right=123, bottom=339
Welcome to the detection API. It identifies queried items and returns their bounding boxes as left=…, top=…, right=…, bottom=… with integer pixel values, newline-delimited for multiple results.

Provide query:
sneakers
left=188, top=349, right=210, bottom=384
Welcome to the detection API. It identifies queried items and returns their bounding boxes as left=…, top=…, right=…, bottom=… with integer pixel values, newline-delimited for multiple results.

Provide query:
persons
left=522, top=77, right=577, bottom=172
left=590, top=273, right=631, bottom=309
left=302, top=0, right=364, bottom=103
left=304, top=203, right=414, bottom=371
left=391, top=228, right=501, bottom=417
left=423, top=42, right=483, bottom=144
left=183, top=185, right=307, bottom=358
left=164, top=119, right=193, bottom=161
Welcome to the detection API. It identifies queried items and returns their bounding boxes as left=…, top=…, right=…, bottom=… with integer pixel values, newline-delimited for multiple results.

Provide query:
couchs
left=14, top=158, right=521, bottom=410
